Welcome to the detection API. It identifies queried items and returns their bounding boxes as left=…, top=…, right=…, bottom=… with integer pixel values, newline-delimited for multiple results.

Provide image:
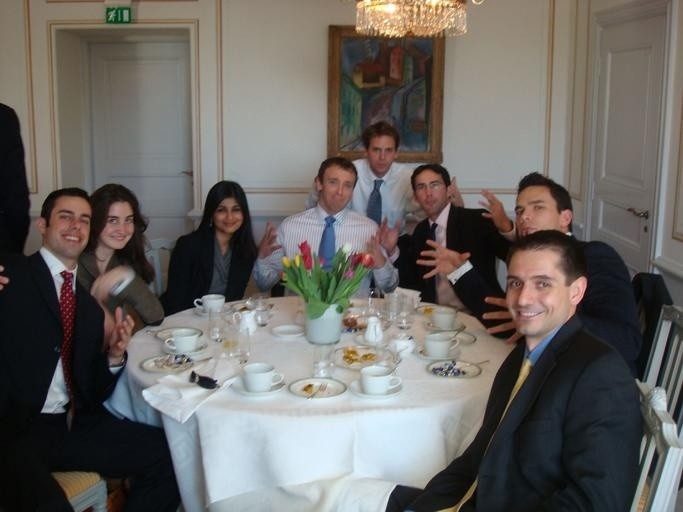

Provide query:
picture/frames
left=324, top=21, right=447, bottom=167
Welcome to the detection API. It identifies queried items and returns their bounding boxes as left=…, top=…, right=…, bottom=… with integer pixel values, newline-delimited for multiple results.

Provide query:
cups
left=194, top=294, right=227, bottom=313
left=360, top=367, right=402, bottom=396
left=432, top=305, right=457, bottom=330
left=313, top=344, right=336, bottom=380
left=209, top=306, right=224, bottom=342
left=364, top=319, right=384, bottom=344
left=425, top=335, right=459, bottom=357
left=166, top=329, right=202, bottom=353
left=242, top=361, right=283, bottom=392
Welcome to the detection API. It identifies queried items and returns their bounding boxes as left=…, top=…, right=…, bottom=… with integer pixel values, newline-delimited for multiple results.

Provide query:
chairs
left=642, top=305, right=682, bottom=435
left=630, top=271, right=674, bottom=377
left=139, top=239, right=177, bottom=294
left=50, top=470, right=107, bottom=511
left=630, top=377, right=683, bottom=512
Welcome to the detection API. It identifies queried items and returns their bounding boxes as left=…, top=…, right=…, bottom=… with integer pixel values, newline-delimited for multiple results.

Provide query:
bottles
left=369, top=291, right=389, bottom=331
left=254, top=290, right=274, bottom=326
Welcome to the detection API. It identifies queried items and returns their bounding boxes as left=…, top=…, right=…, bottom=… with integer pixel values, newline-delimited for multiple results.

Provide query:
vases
left=304, top=298, right=341, bottom=345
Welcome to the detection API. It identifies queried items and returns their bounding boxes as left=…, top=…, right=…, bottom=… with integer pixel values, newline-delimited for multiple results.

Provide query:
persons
left=1, top=183, right=165, bottom=334
left=374, top=163, right=521, bottom=325
left=160, top=179, right=257, bottom=318
left=416, top=170, right=642, bottom=386
left=250, top=156, right=399, bottom=299
left=0, top=187, right=183, bottom=512
left=385, top=228, right=645, bottom=511
left=1, top=103, right=32, bottom=266
left=303, top=119, right=465, bottom=287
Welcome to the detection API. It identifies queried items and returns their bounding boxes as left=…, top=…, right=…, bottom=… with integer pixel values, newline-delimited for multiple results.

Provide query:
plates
left=155, top=325, right=202, bottom=340
left=194, top=304, right=233, bottom=317
left=350, top=377, right=405, bottom=398
left=427, top=318, right=466, bottom=332
left=162, top=339, right=210, bottom=355
left=141, top=353, right=193, bottom=374
left=271, top=325, right=304, bottom=339
left=426, top=359, right=482, bottom=382
left=233, top=374, right=287, bottom=397
left=232, top=300, right=274, bottom=314
left=415, top=304, right=438, bottom=316
left=414, top=342, right=464, bottom=358
left=440, top=327, right=475, bottom=349
left=288, top=376, right=347, bottom=399
left=330, top=343, right=396, bottom=370
left=356, top=334, right=386, bottom=348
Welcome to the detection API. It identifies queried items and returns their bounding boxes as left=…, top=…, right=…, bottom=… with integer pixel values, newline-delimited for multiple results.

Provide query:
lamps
left=349, top=1, right=468, bottom=41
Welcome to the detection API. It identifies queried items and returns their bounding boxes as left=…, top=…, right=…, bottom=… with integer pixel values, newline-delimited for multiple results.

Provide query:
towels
left=140, top=352, right=240, bottom=423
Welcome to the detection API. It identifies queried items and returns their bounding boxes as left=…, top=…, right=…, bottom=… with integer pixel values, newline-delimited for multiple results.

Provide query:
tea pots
left=232, top=310, right=258, bottom=336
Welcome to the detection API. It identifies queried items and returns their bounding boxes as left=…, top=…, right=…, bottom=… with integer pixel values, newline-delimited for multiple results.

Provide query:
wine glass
left=396, top=295, right=416, bottom=338
left=224, top=326, right=250, bottom=369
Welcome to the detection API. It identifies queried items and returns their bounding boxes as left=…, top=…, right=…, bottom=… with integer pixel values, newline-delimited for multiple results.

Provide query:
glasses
left=416, top=183, right=445, bottom=189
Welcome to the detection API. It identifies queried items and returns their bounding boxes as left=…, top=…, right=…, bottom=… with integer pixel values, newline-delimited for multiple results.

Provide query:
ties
left=367, top=180, right=384, bottom=225
left=57, top=270, right=76, bottom=417
left=429, top=223, right=445, bottom=242
left=317, top=216, right=336, bottom=273
left=434, top=358, right=531, bottom=512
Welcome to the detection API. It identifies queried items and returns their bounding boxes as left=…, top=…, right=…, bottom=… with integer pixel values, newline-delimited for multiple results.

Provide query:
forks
left=307, top=379, right=329, bottom=400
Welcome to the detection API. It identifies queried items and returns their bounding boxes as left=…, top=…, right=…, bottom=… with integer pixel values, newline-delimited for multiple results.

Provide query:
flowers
left=274, top=238, right=372, bottom=315
